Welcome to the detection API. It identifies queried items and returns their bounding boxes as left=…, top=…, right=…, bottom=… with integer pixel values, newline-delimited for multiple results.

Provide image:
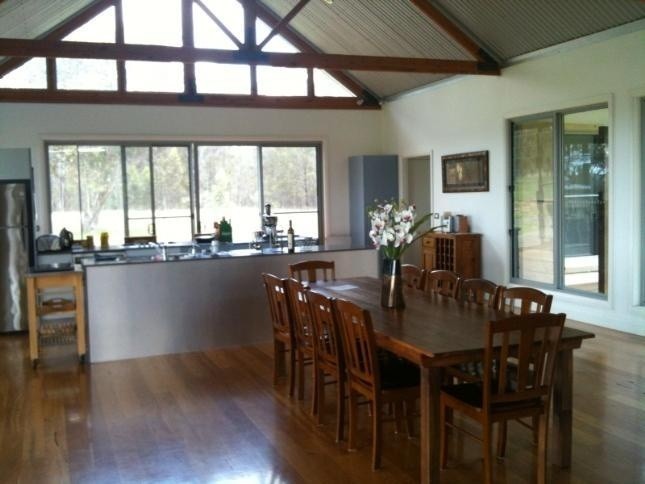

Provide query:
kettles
left=58, top=227, right=74, bottom=252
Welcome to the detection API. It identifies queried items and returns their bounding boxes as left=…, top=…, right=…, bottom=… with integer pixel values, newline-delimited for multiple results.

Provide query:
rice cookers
left=35, top=249, right=74, bottom=272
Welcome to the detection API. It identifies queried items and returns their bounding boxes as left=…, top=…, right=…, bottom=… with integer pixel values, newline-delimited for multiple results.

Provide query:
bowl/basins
left=193, top=234, right=215, bottom=243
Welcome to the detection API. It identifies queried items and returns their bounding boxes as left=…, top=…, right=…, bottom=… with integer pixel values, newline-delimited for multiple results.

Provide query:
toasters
left=34, top=234, right=60, bottom=251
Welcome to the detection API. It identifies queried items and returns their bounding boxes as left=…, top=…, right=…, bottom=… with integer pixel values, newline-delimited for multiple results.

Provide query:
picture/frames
left=441, top=151, right=489, bottom=193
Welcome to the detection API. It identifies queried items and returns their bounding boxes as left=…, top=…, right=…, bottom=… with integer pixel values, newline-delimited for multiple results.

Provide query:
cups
left=209, top=240, right=218, bottom=253
left=454, top=214, right=468, bottom=233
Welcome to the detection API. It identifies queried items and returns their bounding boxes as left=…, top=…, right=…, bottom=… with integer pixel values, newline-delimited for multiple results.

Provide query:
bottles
left=287, top=220, right=294, bottom=254
left=217, top=217, right=232, bottom=242
left=100, top=232, right=109, bottom=249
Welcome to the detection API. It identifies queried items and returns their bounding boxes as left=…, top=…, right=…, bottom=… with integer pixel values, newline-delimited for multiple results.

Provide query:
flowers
left=366, top=195, right=448, bottom=261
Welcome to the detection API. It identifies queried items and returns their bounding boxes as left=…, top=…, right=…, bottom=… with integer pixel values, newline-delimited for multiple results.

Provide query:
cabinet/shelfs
left=26, top=263, right=87, bottom=371
left=420, top=231, right=481, bottom=279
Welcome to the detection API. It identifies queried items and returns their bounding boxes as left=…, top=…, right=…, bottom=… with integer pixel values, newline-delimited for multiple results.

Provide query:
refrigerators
left=0, top=180, right=32, bottom=337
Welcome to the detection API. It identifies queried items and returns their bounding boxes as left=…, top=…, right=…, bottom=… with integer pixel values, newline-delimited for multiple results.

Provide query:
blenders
left=253, top=203, right=278, bottom=250
